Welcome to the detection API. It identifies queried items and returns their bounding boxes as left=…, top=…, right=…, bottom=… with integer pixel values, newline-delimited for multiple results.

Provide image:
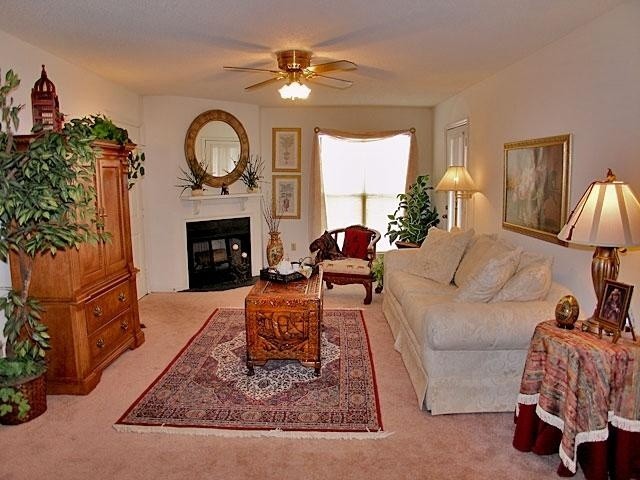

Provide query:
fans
left=222, top=47, right=358, bottom=94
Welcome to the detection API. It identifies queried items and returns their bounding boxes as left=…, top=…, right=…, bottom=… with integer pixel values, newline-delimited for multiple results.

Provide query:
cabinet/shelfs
left=510, top=318, right=638, bottom=479
left=0, top=134, right=146, bottom=396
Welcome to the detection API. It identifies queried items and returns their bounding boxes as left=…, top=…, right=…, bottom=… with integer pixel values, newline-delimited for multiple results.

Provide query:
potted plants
left=384, top=174, right=440, bottom=250
left=0, top=69, right=146, bottom=424
left=223, top=152, right=268, bottom=193
left=175, top=154, right=215, bottom=196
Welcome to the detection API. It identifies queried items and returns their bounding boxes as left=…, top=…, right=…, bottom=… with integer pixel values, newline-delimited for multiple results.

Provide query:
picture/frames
left=270, top=174, right=300, bottom=221
left=596, top=278, right=636, bottom=342
left=270, top=125, right=301, bottom=173
left=501, top=134, right=571, bottom=248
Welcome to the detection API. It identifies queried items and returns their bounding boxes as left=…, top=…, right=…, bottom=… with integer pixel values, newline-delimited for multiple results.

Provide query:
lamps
left=557, top=166, right=639, bottom=336
left=276, top=71, right=312, bottom=101
left=433, top=163, right=479, bottom=230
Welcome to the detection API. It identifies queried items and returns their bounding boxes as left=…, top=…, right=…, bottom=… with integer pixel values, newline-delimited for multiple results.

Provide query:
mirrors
left=184, top=109, right=250, bottom=188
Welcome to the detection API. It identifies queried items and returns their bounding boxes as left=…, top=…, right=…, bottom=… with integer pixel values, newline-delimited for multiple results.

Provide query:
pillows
left=402, top=226, right=556, bottom=303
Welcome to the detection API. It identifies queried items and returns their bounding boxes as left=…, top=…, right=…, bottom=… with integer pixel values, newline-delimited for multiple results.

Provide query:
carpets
left=113, top=308, right=393, bottom=441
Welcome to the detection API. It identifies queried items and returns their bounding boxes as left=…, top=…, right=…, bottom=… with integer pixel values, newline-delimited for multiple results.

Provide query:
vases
left=267, top=231, right=284, bottom=268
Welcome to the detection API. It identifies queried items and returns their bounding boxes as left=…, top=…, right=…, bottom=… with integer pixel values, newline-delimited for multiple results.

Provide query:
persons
left=603, top=288, right=622, bottom=323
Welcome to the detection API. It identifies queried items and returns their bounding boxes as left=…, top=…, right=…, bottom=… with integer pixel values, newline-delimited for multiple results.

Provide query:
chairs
left=309, top=224, right=382, bottom=304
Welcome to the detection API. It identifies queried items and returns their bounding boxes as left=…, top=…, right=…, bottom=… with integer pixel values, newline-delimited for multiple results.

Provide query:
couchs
left=380, top=229, right=585, bottom=418
